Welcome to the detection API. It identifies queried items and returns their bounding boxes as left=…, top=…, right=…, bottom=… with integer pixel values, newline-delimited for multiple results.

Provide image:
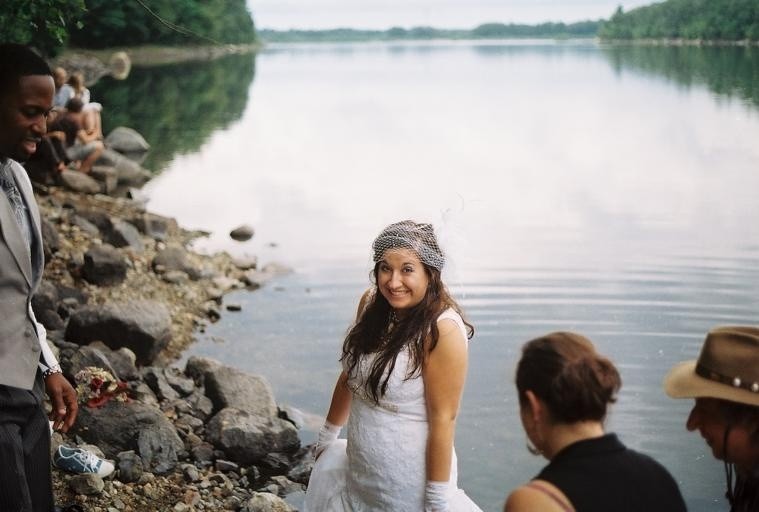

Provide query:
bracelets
left=43, top=367, right=63, bottom=383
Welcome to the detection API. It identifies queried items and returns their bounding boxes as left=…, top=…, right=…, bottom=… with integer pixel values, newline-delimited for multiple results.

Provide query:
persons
left=298, top=219, right=485, bottom=512
left=665, top=322, right=758, bottom=512
left=25, top=65, right=105, bottom=188
left=503, top=330, right=690, bottom=511
left=0, top=42, right=79, bottom=512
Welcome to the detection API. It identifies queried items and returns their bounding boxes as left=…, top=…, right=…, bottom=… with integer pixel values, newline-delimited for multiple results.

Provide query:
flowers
left=73, top=364, right=129, bottom=408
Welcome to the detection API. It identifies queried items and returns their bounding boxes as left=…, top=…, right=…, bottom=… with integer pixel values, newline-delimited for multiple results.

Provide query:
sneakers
left=51, top=445, right=114, bottom=479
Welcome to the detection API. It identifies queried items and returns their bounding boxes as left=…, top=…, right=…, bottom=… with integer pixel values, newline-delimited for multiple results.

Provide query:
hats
left=666, top=325, right=759, bottom=406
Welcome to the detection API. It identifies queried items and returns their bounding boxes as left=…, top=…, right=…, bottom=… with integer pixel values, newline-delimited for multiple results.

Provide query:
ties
left=0, top=164, right=34, bottom=264
